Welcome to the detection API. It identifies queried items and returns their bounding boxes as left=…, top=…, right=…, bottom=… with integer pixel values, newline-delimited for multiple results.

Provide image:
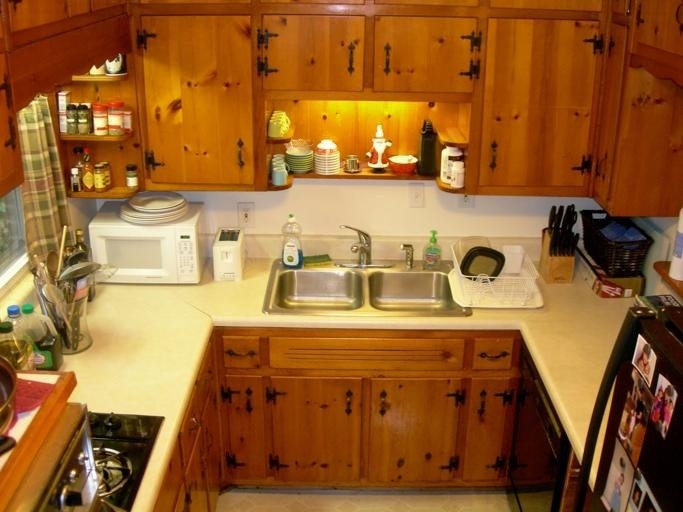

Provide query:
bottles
left=66, top=100, right=133, bottom=137
left=73, top=229, right=96, bottom=301
left=419, top=120, right=465, bottom=190
left=69, top=147, right=139, bottom=194
left=0, top=303, right=64, bottom=371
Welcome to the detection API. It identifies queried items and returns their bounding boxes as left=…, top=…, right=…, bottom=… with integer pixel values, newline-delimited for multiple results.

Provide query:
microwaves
left=88, top=201, right=205, bottom=284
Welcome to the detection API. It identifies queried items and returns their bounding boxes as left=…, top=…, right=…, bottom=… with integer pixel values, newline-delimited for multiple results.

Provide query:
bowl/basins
left=388, top=155, right=418, bottom=174
left=313, top=139, right=341, bottom=176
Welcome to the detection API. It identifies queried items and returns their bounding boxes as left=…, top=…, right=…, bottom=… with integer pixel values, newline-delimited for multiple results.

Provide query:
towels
left=14, top=93, right=76, bottom=277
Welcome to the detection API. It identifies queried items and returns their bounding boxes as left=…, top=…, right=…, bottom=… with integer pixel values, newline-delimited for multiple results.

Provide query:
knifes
left=549, top=204, right=580, bottom=257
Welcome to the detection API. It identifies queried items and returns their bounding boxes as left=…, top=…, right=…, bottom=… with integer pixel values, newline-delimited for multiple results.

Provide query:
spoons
left=47, top=251, right=59, bottom=281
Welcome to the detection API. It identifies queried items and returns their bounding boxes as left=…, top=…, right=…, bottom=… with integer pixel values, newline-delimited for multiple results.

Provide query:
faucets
left=340, top=225, right=371, bottom=265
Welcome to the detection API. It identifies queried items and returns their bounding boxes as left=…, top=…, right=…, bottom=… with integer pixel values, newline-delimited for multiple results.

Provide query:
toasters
left=212, top=227, right=244, bottom=282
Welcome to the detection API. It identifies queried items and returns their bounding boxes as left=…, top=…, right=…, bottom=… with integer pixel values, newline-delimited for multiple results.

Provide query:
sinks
left=262, top=258, right=366, bottom=317
left=365, top=260, right=472, bottom=317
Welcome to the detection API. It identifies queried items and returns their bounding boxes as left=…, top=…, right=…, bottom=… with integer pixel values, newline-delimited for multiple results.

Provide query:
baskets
left=579, top=209, right=654, bottom=277
left=452, top=239, right=539, bottom=306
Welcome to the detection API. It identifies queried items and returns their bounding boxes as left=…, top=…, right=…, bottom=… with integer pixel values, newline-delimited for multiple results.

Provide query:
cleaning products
left=281, top=212, right=304, bottom=269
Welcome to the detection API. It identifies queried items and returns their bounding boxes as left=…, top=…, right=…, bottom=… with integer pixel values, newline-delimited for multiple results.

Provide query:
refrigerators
left=574, top=304, right=683, bottom=512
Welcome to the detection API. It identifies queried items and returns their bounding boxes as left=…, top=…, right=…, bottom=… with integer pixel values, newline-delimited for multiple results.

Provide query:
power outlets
left=237, top=201, right=254, bottom=229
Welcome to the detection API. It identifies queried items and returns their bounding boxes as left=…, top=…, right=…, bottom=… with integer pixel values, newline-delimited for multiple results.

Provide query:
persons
left=644, top=506, right=654, bottom=512
left=623, top=378, right=648, bottom=468
left=612, top=473, right=626, bottom=512
left=651, top=384, right=674, bottom=439
left=634, top=342, right=653, bottom=384
left=631, top=489, right=641, bottom=507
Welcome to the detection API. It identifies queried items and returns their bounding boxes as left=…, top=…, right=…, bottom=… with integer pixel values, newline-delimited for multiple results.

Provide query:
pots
left=0, top=352, right=15, bottom=436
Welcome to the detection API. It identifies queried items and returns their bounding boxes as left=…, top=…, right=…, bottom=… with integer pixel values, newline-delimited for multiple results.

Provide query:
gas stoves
left=88, top=412, right=166, bottom=512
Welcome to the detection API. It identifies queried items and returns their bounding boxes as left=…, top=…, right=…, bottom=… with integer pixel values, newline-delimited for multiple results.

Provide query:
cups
left=271, top=154, right=289, bottom=186
left=343, top=155, right=360, bottom=173
left=267, top=110, right=290, bottom=138
left=38, top=295, right=91, bottom=355
left=504, top=245, right=524, bottom=274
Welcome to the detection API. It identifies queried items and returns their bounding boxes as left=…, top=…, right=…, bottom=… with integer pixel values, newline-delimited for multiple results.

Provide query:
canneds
left=94, top=163, right=107, bottom=192
left=100, top=162, right=112, bottom=188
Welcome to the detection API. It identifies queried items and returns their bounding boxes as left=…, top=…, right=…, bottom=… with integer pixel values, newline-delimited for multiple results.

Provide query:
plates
left=460, top=245, right=506, bottom=282
left=118, top=191, right=189, bottom=225
left=285, top=148, right=313, bottom=175
